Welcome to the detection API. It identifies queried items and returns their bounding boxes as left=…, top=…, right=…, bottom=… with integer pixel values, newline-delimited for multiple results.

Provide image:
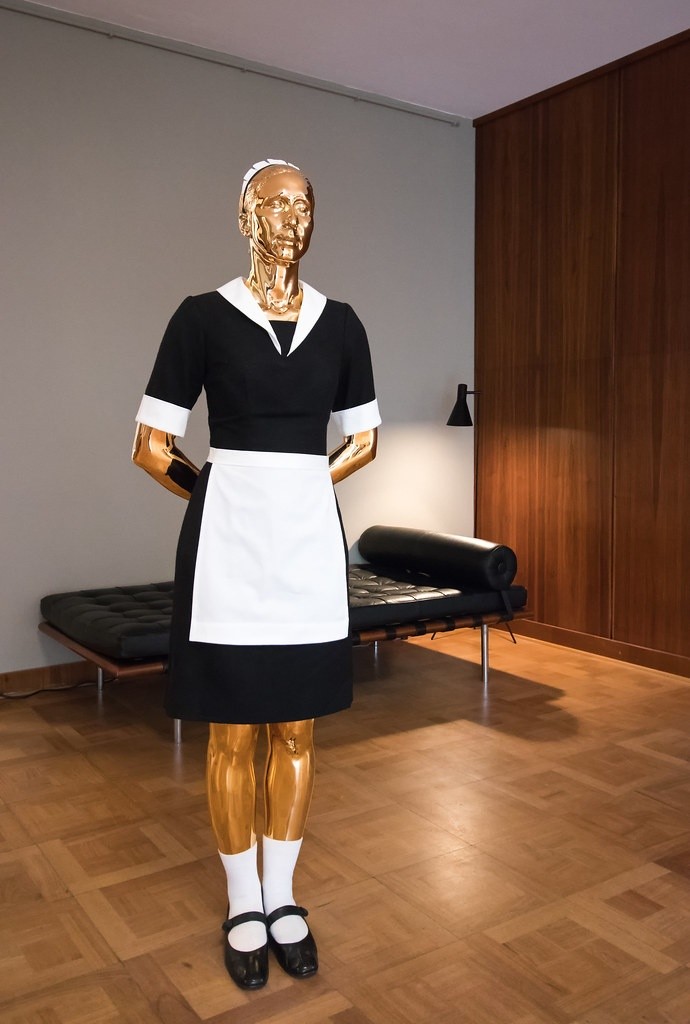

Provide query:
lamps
left=447, top=384, right=481, bottom=426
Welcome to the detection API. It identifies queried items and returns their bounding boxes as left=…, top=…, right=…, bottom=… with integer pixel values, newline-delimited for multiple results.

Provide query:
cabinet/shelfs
left=463, top=29, right=690, bottom=678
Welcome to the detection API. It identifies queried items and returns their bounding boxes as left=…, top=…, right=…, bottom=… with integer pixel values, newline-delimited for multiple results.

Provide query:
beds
left=42, top=525, right=529, bottom=744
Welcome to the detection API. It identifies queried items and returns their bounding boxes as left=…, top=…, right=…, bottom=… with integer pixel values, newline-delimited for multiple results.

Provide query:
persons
left=132, top=159, right=382, bottom=990
